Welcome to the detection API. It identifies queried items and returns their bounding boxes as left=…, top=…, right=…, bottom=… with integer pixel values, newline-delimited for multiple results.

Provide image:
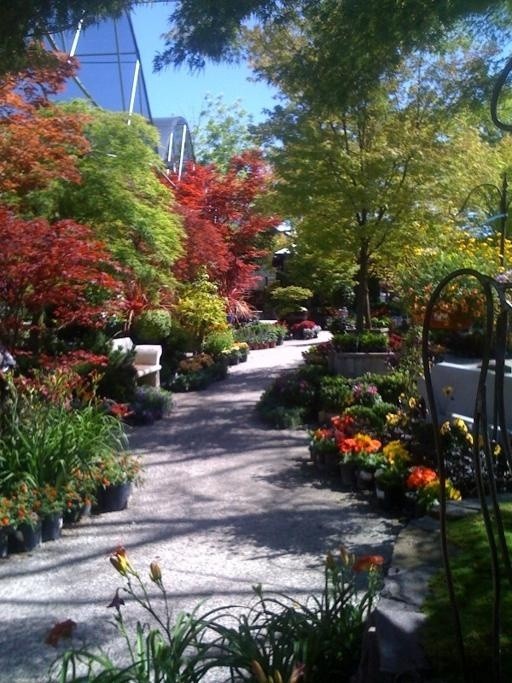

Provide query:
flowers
left=307, top=415, right=461, bottom=507
left=221, top=321, right=335, bottom=367
left=0, top=446, right=138, bottom=534
left=110, top=392, right=166, bottom=427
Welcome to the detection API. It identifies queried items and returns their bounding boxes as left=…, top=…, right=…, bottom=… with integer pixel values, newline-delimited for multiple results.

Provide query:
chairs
left=108, top=336, right=163, bottom=389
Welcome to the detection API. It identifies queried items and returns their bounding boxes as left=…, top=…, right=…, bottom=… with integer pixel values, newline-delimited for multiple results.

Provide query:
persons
left=0, top=340, right=16, bottom=362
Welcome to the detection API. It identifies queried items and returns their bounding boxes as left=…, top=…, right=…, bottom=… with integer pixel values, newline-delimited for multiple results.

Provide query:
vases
left=6, top=513, right=63, bottom=552
left=375, top=488, right=402, bottom=518
